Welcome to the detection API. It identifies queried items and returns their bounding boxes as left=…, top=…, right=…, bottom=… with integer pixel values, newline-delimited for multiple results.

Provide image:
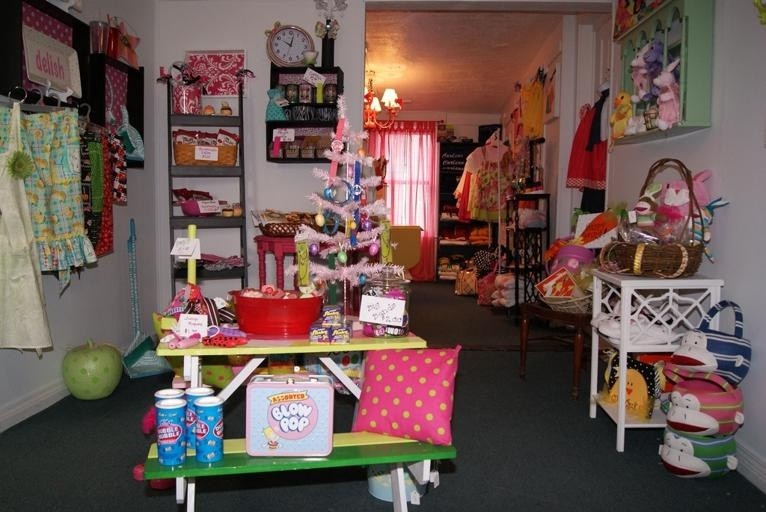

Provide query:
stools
left=517, top=298, right=592, bottom=398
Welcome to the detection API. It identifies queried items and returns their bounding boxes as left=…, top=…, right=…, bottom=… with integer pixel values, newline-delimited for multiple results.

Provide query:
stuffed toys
left=632, top=170, right=731, bottom=263
left=611, top=36, right=680, bottom=139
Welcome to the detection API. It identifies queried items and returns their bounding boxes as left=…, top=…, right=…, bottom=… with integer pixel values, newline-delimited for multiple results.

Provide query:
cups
left=171, top=85, right=203, bottom=115
left=222, top=208, right=233, bottom=218
left=233, top=202, right=241, bottom=218
left=276, top=84, right=336, bottom=104
left=109, top=26, right=120, bottom=58
left=160, top=65, right=171, bottom=79
left=89, top=21, right=111, bottom=55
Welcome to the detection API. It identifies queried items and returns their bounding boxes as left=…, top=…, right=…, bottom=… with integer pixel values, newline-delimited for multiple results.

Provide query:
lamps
left=364, top=69, right=406, bottom=131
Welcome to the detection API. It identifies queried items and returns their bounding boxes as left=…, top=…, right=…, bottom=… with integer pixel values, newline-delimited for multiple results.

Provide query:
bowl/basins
left=228, top=290, right=325, bottom=340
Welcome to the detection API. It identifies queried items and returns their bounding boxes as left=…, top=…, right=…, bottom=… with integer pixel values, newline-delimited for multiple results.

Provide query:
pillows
left=349, top=342, right=469, bottom=447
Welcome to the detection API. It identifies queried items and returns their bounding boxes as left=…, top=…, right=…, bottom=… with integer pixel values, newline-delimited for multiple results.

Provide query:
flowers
left=311, top=0, right=349, bottom=41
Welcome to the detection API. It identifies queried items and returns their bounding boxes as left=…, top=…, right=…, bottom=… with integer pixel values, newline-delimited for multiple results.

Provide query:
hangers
left=4, top=85, right=112, bottom=146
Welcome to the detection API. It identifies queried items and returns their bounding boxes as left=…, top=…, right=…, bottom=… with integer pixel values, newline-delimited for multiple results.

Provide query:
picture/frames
left=543, top=57, right=561, bottom=126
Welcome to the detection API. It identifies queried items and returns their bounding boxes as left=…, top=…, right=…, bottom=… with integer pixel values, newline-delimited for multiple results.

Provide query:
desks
left=253, top=233, right=384, bottom=316
left=387, top=224, right=426, bottom=283
left=155, top=328, right=431, bottom=511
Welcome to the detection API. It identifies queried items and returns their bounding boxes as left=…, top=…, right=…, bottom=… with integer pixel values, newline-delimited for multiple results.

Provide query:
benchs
left=141, top=429, right=459, bottom=511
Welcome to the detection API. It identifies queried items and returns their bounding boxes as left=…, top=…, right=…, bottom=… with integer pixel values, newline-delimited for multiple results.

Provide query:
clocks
left=263, top=20, right=314, bottom=68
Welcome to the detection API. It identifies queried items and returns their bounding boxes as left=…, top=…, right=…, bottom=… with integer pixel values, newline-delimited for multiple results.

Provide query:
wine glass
left=302, top=49, right=319, bottom=68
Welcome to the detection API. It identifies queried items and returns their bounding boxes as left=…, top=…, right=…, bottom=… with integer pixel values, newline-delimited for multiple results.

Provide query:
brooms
left=122, top=217, right=155, bottom=368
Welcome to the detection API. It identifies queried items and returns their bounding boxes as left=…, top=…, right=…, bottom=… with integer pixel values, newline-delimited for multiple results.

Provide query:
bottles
left=221, top=108, right=232, bottom=115
left=202, top=105, right=214, bottom=116
left=644, top=108, right=658, bottom=130
left=271, top=136, right=332, bottom=159
left=218, top=200, right=228, bottom=217
left=362, top=267, right=409, bottom=338
left=152, top=387, right=224, bottom=465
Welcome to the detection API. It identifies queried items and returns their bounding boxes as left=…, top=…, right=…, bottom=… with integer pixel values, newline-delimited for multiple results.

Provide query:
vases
left=321, top=30, right=335, bottom=68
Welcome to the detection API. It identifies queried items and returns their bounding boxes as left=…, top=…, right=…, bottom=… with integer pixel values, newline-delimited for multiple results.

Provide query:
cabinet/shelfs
left=265, top=61, right=345, bottom=165
left=611, top=1, right=717, bottom=146
left=504, top=192, right=553, bottom=321
left=165, top=73, right=249, bottom=308
left=82, top=52, right=149, bottom=173
left=0, top=0, right=94, bottom=117
left=435, top=141, right=500, bottom=286
left=587, top=266, right=726, bottom=453
